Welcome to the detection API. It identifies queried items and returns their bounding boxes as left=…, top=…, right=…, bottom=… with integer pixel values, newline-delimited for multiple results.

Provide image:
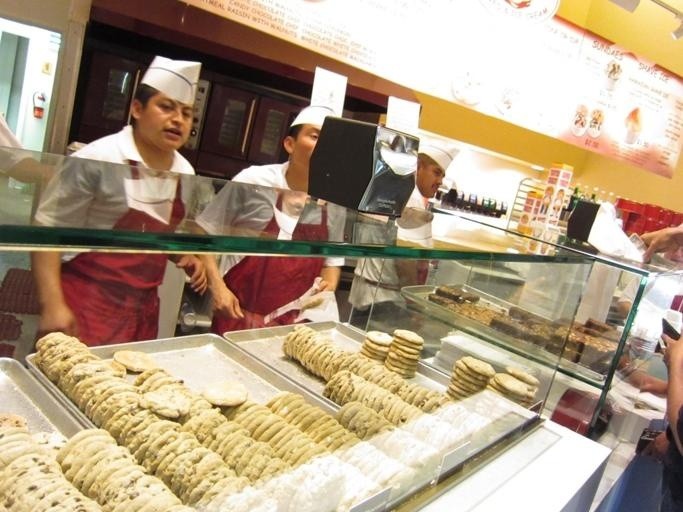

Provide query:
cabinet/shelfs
left=365, top=206, right=683, bottom=512
left=2, top=174, right=612, bottom=512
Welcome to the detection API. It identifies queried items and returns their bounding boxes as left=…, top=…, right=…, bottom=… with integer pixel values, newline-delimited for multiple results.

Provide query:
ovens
left=66, top=22, right=312, bottom=181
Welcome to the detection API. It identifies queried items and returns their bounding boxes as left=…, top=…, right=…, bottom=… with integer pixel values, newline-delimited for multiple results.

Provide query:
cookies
left=506, top=366, right=540, bottom=409
left=282, top=323, right=450, bottom=428
left=486, top=373, right=528, bottom=407
left=445, top=355, right=496, bottom=406
left=0, top=408, right=194, bottom=512
left=34, top=332, right=397, bottom=510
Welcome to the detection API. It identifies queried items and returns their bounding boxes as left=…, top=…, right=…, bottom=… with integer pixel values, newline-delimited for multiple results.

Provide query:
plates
left=1, top=357, right=84, bottom=445
left=222, top=318, right=452, bottom=412
left=24, top=332, right=339, bottom=428
left=400, top=283, right=626, bottom=381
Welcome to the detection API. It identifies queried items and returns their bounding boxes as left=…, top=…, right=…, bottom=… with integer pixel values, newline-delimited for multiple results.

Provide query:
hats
left=418, top=138, right=460, bottom=171
left=141, top=54, right=202, bottom=106
left=289, top=90, right=339, bottom=126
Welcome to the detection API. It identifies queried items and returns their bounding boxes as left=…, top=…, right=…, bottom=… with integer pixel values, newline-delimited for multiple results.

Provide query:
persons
left=0, top=111, right=58, bottom=187
left=193, top=105, right=348, bottom=336
left=635, top=222, right=683, bottom=463
left=30, top=55, right=208, bottom=349
left=659, top=329, right=683, bottom=512
left=349, top=142, right=460, bottom=330
